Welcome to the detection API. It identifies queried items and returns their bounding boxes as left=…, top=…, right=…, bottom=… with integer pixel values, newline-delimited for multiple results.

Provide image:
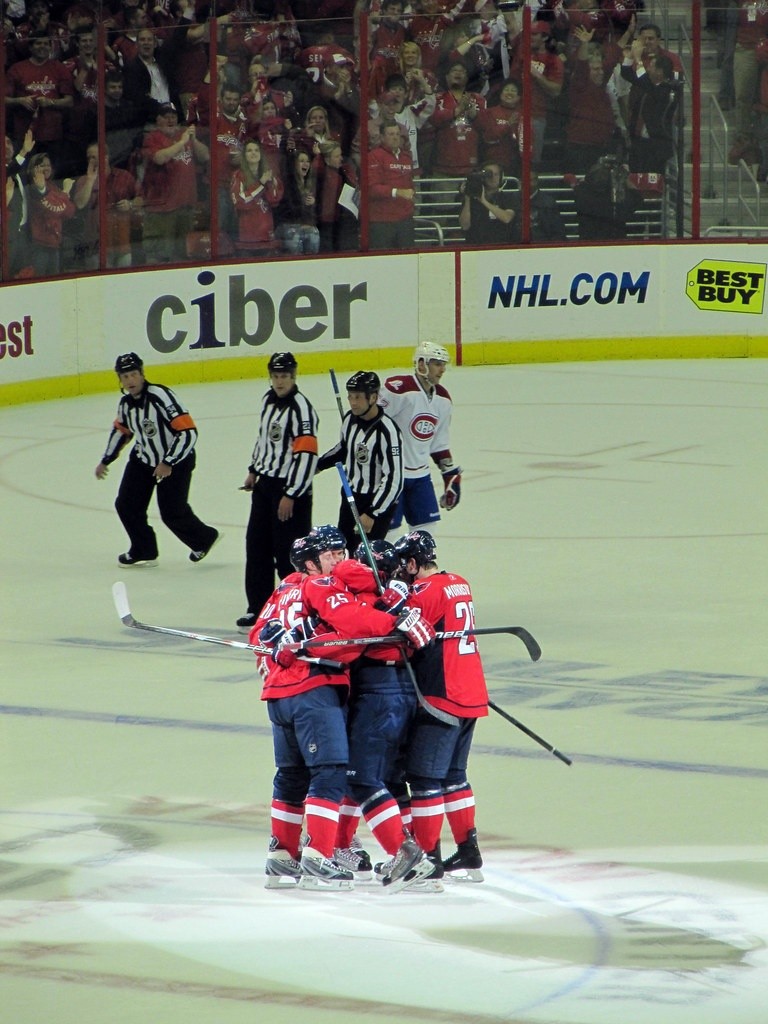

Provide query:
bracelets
left=391, top=187, right=397, bottom=198
left=50, top=99, right=56, bottom=107
left=347, top=90, right=353, bottom=94
left=636, top=60, right=644, bottom=65
left=467, top=40, right=473, bottom=47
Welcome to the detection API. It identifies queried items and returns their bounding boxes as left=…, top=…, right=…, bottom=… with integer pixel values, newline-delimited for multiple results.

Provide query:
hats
left=379, top=91, right=400, bottom=106
left=324, top=53, right=353, bottom=67
left=159, top=102, right=177, bottom=116
left=531, top=20, right=550, bottom=34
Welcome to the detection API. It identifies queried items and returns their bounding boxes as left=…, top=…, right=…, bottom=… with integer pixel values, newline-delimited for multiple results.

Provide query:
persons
left=315, top=372, right=404, bottom=559
left=0, top=0, right=768, bottom=285
left=375, top=340, right=463, bottom=541
left=236, top=351, right=320, bottom=634
left=94, top=351, right=225, bottom=570
left=248, top=526, right=490, bottom=893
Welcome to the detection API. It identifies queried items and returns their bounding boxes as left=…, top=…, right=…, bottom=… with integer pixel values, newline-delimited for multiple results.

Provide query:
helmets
left=415, top=341, right=450, bottom=363
left=268, top=351, right=297, bottom=372
left=309, top=524, right=347, bottom=550
left=115, top=352, right=143, bottom=373
left=346, top=371, right=380, bottom=392
left=354, top=540, right=400, bottom=573
left=394, top=530, right=436, bottom=556
left=290, top=532, right=331, bottom=565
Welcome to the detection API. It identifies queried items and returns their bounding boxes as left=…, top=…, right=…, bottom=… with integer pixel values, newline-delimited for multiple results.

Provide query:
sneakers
left=236, top=613, right=258, bottom=635
left=118, top=548, right=160, bottom=568
left=264, top=824, right=485, bottom=894
left=189, top=528, right=222, bottom=563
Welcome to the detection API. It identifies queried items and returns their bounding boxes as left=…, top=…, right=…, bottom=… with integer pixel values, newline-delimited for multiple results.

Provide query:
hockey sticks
left=112, top=582, right=343, bottom=669
left=335, top=462, right=460, bottom=727
left=329, top=368, right=344, bottom=418
left=280, top=626, right=542, bottom=662
left=488, top=701, right=573, bottom=766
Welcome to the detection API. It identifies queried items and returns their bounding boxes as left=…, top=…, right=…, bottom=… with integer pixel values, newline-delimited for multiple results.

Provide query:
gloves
left=271, top=627, right=304, bottom=668
left=439, top=465, right=464, bottom=511
left=396, top=610, right=436, bottom=651
left=373, top=578, right=409, bottom=615
left=259, top=619, right=287, bottom=648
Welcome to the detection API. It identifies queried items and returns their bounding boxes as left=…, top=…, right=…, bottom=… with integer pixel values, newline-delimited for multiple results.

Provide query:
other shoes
left=757, top=174, right=766, bottom=181
left=720, top=97, right=729, bottom=110
